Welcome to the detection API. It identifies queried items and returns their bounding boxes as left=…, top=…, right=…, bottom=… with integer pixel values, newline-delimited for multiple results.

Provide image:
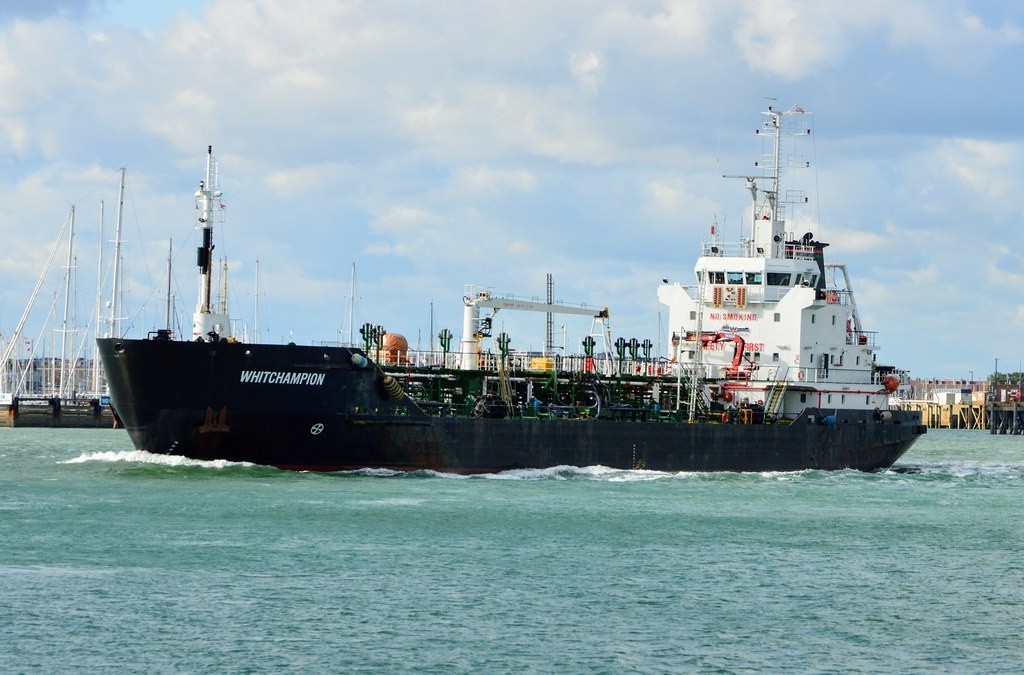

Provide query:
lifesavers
left=798, top=370, right=805, bottom=380
left=722, top=412, right=730, bottom=424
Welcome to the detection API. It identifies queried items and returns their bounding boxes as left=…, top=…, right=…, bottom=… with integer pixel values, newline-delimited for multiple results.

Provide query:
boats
left=1, top=102, right=931, bottom=476
